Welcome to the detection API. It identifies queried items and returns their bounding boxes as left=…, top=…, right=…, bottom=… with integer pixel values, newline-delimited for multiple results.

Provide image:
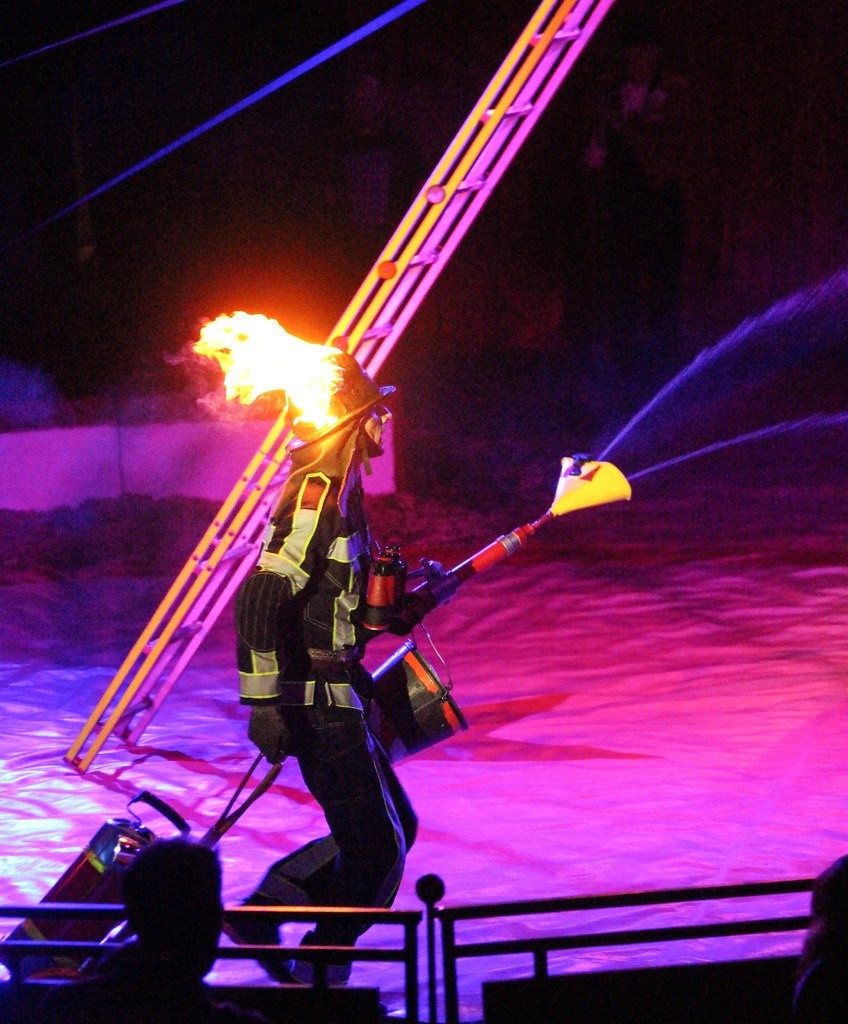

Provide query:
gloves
left=246, top=702, right=289, bottom=766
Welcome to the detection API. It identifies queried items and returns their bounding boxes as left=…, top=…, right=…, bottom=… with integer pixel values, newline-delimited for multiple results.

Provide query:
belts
left=295, top=647, right=364, bottom=671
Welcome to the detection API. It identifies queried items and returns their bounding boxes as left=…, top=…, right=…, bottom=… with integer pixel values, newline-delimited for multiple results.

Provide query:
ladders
left=63, top=0, right=615, bottom=774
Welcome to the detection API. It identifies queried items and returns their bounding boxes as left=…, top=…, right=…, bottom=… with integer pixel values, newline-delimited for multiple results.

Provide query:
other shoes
left=223, top=891, right=291, bottom=984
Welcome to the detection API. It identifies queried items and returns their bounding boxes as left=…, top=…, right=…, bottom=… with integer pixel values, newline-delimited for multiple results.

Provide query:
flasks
left=364, top=552, right=393, bottom=633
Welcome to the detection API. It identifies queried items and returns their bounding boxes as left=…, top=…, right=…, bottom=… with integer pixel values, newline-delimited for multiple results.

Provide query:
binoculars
left=362, top=543, right=408, bottom=633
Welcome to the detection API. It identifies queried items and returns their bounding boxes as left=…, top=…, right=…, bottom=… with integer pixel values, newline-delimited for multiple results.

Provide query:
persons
left=316, top=72, right=421, bottom=234
left=36, top=840, right=268, bottom=1024
left=793, top=855, right=848, bottom=1024
left=221, top=354, right=418, bottom=983
left=563, top=60, right=655, bottom=351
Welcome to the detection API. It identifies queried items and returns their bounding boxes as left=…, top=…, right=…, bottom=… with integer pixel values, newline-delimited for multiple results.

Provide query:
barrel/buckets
left=365, top=621, right=468, bottom=763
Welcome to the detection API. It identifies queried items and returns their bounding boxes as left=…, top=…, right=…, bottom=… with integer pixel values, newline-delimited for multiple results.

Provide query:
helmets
left=286, top=352, right=397, bottom=452
